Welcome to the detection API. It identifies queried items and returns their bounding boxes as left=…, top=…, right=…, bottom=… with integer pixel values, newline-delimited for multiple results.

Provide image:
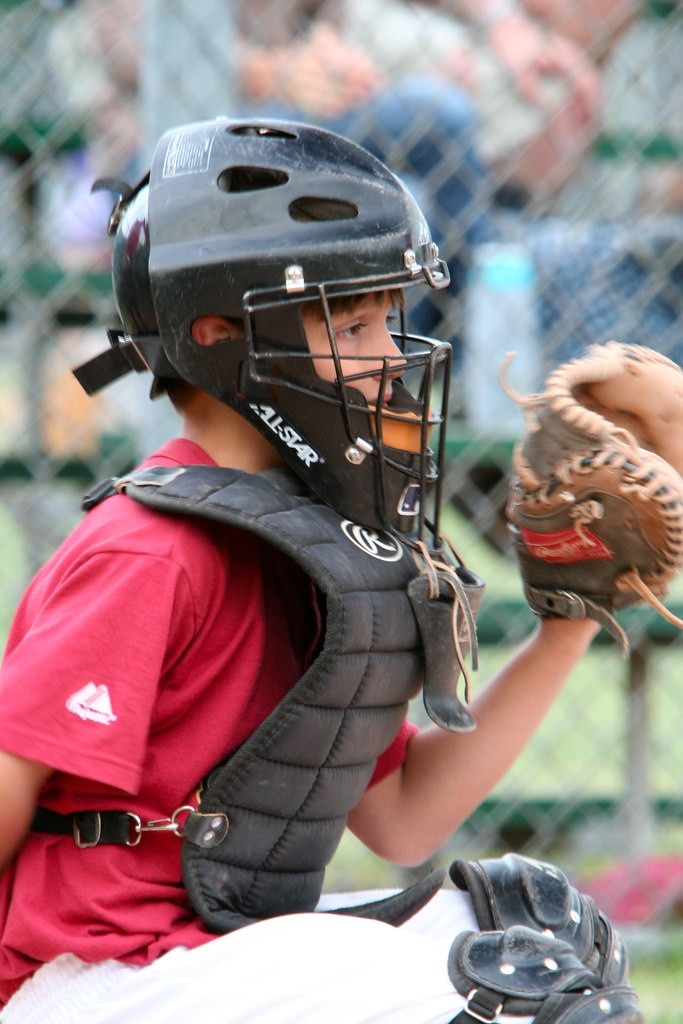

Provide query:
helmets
left=72, top=118, right=454, bottom=548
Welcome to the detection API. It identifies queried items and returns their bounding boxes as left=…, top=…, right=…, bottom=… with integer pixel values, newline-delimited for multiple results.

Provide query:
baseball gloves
left=509, top=338, right=683, bottom=615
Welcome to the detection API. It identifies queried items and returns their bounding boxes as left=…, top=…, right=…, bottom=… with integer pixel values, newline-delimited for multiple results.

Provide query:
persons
left=0, top=116, right=683, bottom=1024
left=48, top=0, right=683, bottom=382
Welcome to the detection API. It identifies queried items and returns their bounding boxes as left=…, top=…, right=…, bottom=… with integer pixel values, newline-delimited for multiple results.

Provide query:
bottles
left=461, top=245, right=543, bottom=440
